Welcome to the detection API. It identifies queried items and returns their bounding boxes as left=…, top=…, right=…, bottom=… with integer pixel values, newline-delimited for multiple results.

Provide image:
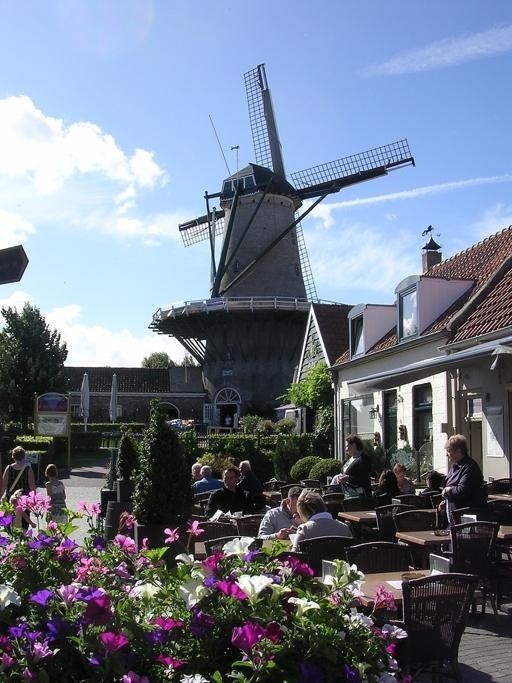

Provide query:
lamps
left=369, top=404, right=378, bottom=418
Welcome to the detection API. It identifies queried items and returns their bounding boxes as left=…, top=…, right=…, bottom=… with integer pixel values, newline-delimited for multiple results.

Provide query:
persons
left=1, top=446, right=68, bottom=531
left=441, top=434, right=487, bottom=531
left=191, top=436, right=442, bottom=552
